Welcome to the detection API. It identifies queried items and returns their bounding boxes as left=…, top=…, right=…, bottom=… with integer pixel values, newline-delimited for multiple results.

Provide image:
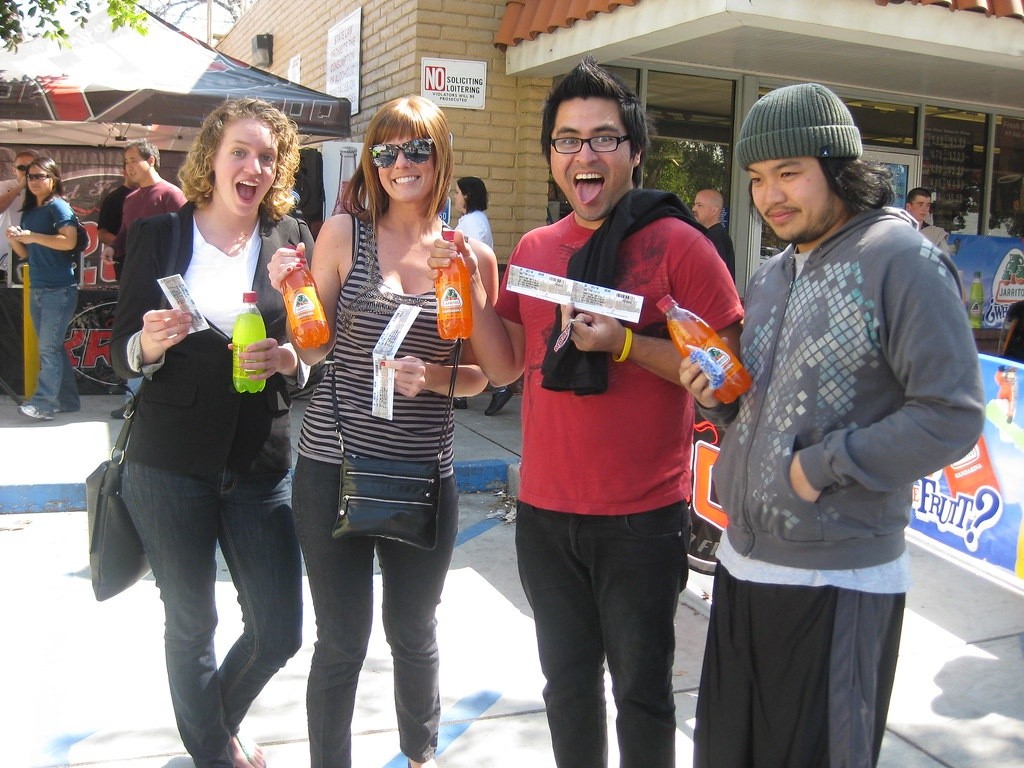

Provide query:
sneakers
left=18, top=404, right=54, bottom=419
left=485, top=387, right=513, bottom=415
left=454, top=394, right=468, bottom=409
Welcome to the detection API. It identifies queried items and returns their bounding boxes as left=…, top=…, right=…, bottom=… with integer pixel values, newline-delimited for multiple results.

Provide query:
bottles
left=969, top=271, right=984, bottom=329
left=435, top=230, right=473, bottom=339
left=656, top=294, right=752, bottom=405
left=281, top=244, right=330, bottom=350
left=331, top=146, right=361, bottom=217
left=232, top=291, right=267, bottom=393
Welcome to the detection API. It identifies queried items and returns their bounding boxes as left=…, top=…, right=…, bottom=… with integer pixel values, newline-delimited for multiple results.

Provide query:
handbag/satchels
left=332, top=456, right=441, bottom=547
left=86, top=449, right=153, bottom=599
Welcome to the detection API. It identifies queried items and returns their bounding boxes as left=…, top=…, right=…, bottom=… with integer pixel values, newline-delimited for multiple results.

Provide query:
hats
left=736, top=83, right=863, bottom=170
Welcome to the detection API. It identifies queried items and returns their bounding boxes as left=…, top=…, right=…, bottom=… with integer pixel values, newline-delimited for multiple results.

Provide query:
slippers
left=230, top=733, right=267, bottom=768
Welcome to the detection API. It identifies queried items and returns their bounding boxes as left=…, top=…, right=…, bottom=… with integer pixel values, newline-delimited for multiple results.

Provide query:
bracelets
left=612, top=328, right=633, bottom=362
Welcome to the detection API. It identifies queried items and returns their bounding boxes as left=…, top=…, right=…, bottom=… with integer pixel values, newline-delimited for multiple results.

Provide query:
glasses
left=14, top=164, right=31, bottom=172
left=551, top=134, right=632, bottom=154
left=368, top=138, right=434, bottom=168
left=26, top=174, right=52, bottom=182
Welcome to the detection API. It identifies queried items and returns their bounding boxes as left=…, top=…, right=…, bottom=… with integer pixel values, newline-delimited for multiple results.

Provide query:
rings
left=571, top=319, right=584, bottom=323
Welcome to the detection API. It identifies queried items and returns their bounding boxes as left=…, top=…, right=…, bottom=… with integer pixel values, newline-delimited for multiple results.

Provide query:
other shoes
left=112, top=405, right=127, bottom=418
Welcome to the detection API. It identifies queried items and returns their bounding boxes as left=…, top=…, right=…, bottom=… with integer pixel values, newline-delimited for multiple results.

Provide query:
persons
left=109, top=96, right=328, bottom=768
left=692, top=189, right=735, bottom=283
left=267, top=93, right=499, bottom=768
left=96, top=160, right=134, bottom=279
left=0, top=150, right=42, bottom=281
left=427, top=58, right=745, bottom=768
left=6, top=157, right=82, bottom=419
left=905, top=187, right=948, bottom=253
left=109, top=139, right=187, bottom=419
left=679, top=83, right=986, bottom=768
left=454, top=176, right=512, bottom=416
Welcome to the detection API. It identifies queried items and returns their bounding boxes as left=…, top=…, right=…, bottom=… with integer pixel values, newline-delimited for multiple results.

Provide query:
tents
left=0, top=0, right=351, bottom=145
